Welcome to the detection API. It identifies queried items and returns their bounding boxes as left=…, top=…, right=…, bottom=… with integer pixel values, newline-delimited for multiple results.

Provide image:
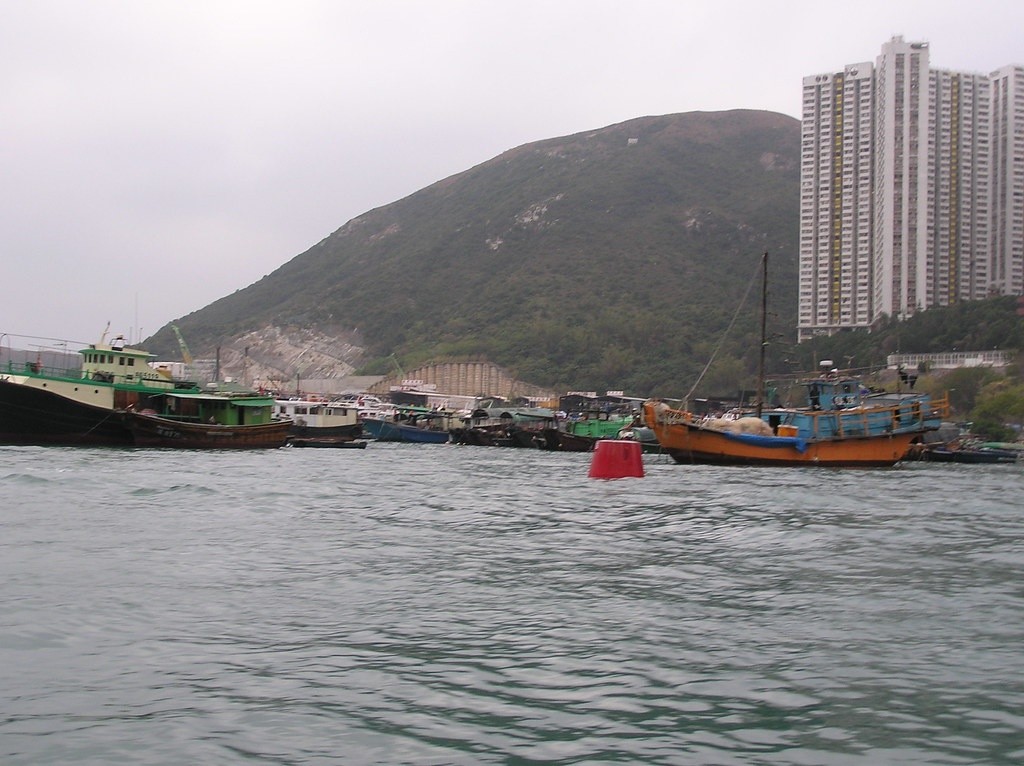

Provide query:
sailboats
left=643, top=252, right=950, bottom=468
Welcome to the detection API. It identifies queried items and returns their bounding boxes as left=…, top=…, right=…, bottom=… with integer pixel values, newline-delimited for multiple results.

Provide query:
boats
left=928, top=440, right=1017, bottom=464
left=272, top=394, right=668, bottom=455
left=0, top=320, right=295, bottom=449
